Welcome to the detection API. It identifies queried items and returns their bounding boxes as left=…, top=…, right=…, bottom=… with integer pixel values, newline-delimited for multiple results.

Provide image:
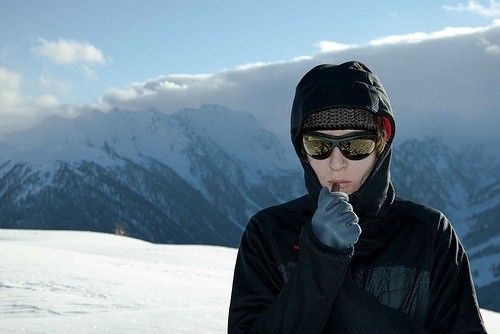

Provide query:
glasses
left=301, top=130, right=379, bottom=160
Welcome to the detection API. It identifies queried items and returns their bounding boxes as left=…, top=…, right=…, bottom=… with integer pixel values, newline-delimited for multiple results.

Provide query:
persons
left=228, top=61, right=487, bottom=334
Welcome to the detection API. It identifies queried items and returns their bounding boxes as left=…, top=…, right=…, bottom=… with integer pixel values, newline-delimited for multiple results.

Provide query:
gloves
left=312, top=187, right=362, bottom=249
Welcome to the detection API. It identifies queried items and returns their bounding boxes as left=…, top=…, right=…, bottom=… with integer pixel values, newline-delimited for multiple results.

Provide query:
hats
left=302, top=108, right=381, bottom=132
